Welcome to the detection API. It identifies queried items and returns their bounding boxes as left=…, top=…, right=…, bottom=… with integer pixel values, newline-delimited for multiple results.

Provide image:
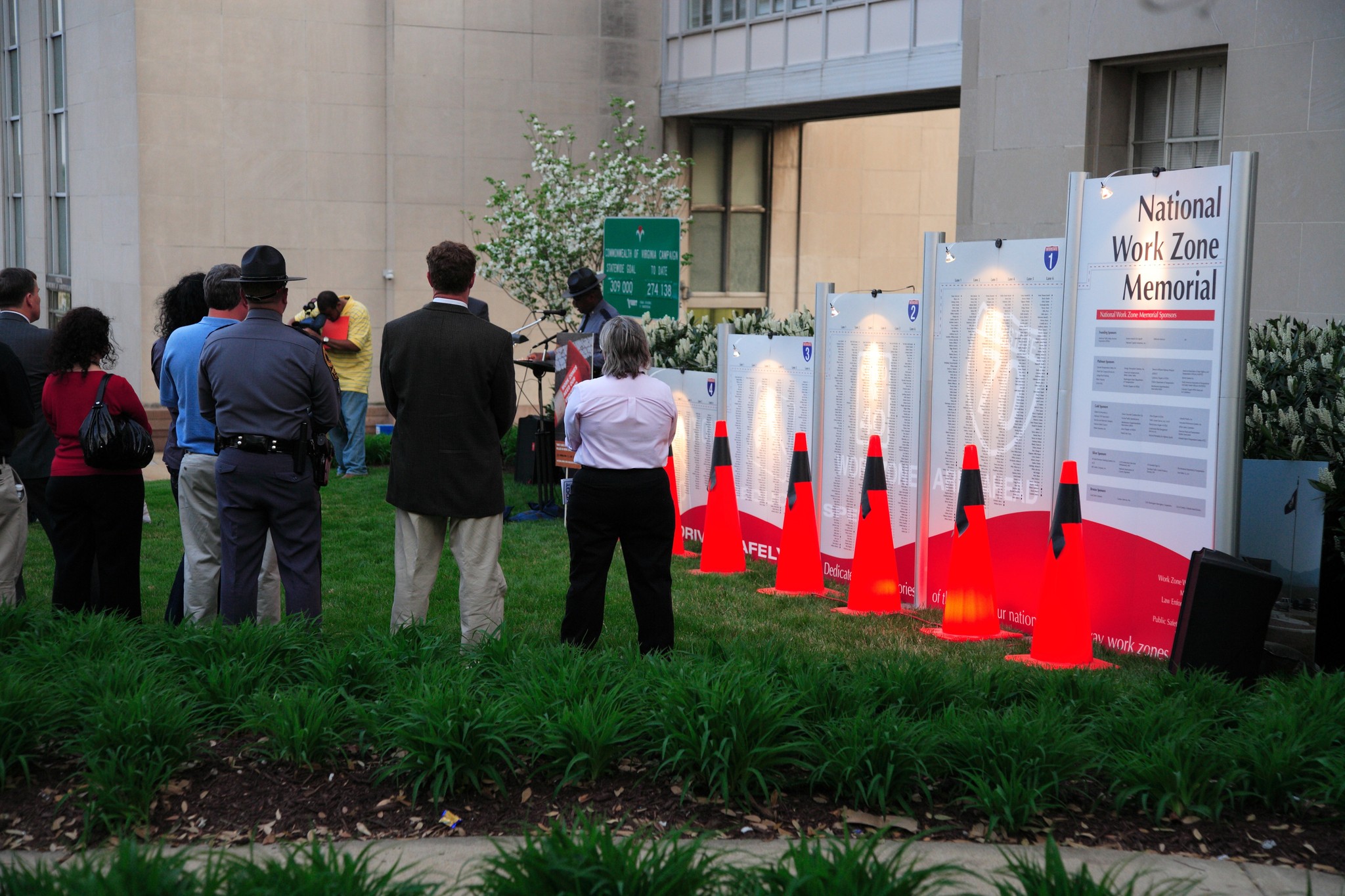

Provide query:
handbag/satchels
left=79, top=374, right=154, bottom=470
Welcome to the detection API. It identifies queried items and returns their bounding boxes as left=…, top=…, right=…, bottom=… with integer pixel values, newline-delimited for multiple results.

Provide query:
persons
left=0, top=268, right=57, bottom=608
left=284, top=290, right=373, bottom=479
left=558, top=318, right=675, bottom=659
left=156, top=264, right=284, bottom=628
left=198, top=246, right=341, bottom=637
left=525, top=265, right=621, bottom=382
left=379, top=240, right=516, bottom=649
left=151, top=273, right=210, bottom=627
left=41, top=306, right=155, bottom=626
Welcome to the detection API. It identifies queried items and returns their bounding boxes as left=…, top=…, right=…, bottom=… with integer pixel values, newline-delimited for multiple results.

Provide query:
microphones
left=532, top=329, right=569, bottom=348
left=532, top=309, right=566, bottom=316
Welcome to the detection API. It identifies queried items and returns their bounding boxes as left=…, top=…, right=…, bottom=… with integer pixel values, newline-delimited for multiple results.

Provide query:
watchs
left=322, top=336, right=329, bottom=345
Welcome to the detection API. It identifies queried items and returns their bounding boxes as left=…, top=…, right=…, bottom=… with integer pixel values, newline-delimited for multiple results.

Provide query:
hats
left=561, top=267, right=606, bottom=299
left=220, top=246, right=307, bottom=283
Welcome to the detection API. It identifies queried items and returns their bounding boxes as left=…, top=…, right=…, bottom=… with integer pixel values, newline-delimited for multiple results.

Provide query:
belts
left=226, top=436, right=297, bottom=455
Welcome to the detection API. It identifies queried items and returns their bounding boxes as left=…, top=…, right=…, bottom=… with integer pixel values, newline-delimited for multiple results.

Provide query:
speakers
left=512, top=415, right=558, bottom=484
left=1166, top=547, right=1283, bottom=695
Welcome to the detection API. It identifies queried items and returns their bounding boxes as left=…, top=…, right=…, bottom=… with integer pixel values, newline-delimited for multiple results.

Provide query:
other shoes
left=142, top=503, right=152, bottom=523
left=343, top=473, right=368, bottom=478
